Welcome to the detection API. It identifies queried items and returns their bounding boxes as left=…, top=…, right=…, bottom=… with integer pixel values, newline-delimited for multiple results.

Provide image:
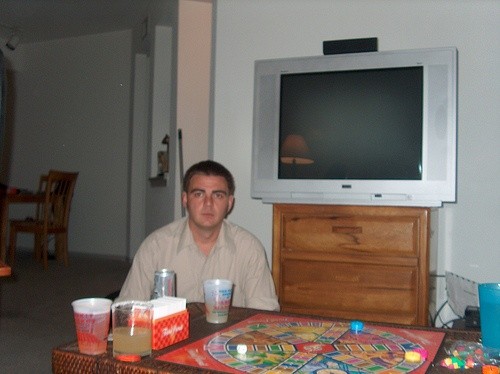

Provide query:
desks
left=0, top=187, right=59, bottom=264
left=50, top=300, right=500, bottom=374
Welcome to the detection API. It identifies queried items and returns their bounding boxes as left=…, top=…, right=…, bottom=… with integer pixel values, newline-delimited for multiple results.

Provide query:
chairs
left=8, top=168, right=80, bottom=270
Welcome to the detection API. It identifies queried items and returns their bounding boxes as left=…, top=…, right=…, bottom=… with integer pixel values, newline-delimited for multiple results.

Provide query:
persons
left=111, top=160, right=281, bottom=312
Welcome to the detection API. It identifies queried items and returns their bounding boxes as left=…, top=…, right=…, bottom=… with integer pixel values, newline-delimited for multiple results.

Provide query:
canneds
left=152, top=268, right=176, bottom=298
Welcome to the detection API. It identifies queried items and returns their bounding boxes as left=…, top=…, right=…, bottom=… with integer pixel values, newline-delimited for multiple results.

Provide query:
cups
left=112, top=300, right=153, bottom=362
left=478, top=283, right=500, bottom=366
left=202, top=278, right=233, bottom=323
left=71, top=298, right=112, bottom=355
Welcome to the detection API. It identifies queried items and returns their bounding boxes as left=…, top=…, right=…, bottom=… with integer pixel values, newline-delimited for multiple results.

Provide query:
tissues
left=128, top=296, right=190, bottom=351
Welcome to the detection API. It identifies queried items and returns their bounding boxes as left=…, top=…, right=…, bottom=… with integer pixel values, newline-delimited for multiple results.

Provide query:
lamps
left=6, top=27, right=24, bottom=51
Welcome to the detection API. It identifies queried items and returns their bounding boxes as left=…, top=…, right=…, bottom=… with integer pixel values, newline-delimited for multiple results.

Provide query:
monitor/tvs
left=252, top=47, right=458, bottom=208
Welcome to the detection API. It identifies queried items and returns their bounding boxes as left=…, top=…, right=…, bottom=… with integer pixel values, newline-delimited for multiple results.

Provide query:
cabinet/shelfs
left=270, top=203, right=431, bottom=330
